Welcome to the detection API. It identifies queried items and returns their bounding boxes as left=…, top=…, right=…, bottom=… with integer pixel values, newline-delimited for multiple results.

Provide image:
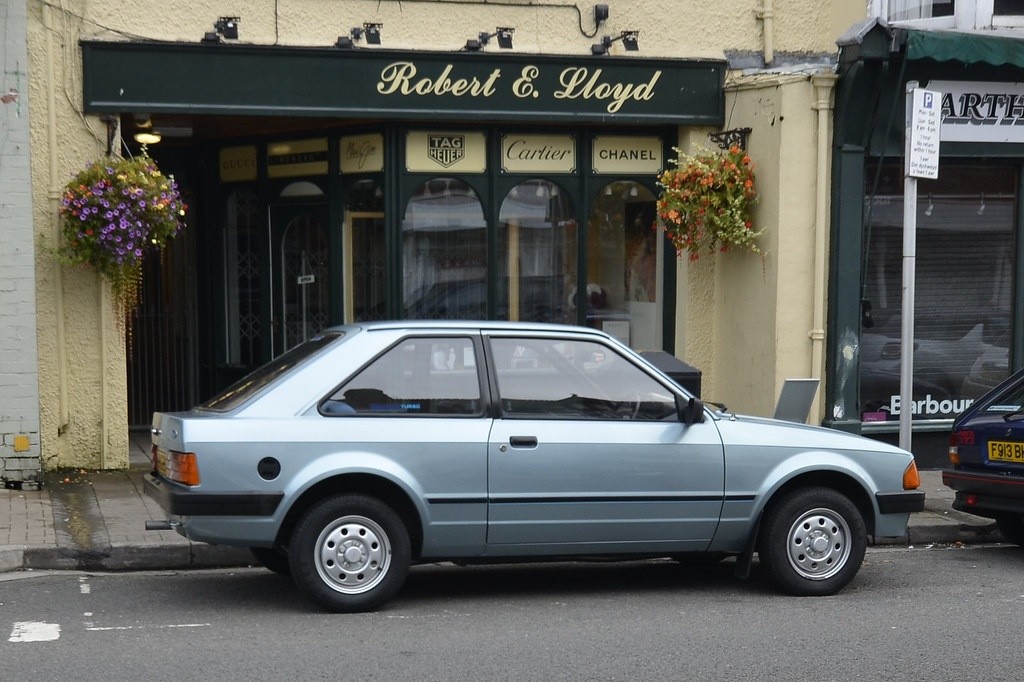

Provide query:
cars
left=857, top=314, right=1017, bottom=406
left=140, top=320, right=925, bottom=614
left=942, top=365, right=1024, bottom=545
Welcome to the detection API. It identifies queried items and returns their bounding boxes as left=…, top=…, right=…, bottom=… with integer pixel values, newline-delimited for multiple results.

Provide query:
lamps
left=200, top=15, right=242, bottom=45
left=464, top=26, right=515, bottom=51
left=590, top=30, right=639, bottom=56
left=336, top=22, right=383, bottom=47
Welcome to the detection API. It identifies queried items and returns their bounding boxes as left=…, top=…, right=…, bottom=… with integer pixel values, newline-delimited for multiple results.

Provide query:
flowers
left=55, top=153, right=189, bottom=360
left=652, top=143, right=768, bottom=259
